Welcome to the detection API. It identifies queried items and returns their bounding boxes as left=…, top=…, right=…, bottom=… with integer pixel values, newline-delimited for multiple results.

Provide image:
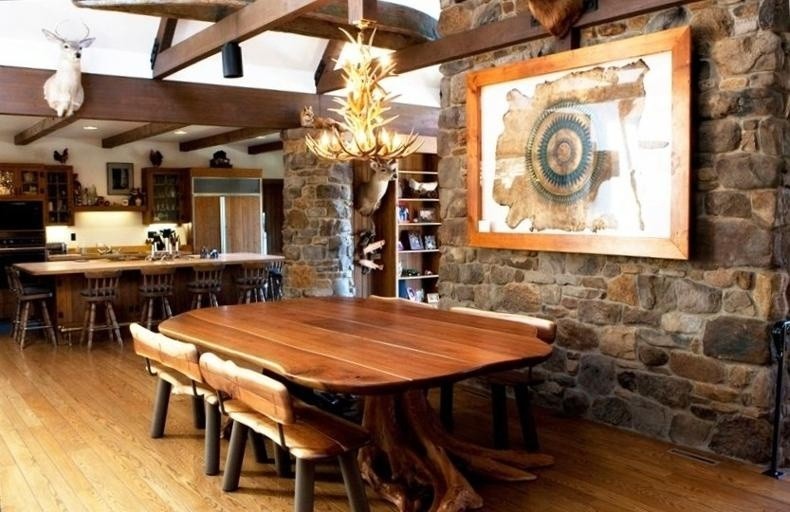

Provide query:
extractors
left=0, top=200, right=43, bottom=229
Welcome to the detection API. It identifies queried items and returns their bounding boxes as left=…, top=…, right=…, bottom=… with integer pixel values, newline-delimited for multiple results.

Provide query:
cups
left=96, top=243, right=104, bottom=251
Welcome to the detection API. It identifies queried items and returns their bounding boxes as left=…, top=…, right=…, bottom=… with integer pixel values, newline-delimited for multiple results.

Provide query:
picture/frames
left=107, top=162, right=134, bottom=195
left=463, top=26, right=691, bottom=262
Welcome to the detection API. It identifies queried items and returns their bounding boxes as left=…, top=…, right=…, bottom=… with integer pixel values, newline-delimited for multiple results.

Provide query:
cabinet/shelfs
left=142, top=167, right=192, bottom=224
left=373, top=153, right=439, bottom=297
left=0, top=163, right=74, bottom=324
left=192, top=167, right=264, bottom=254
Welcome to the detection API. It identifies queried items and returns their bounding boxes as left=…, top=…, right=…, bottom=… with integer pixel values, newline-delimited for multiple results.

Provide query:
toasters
left=48, top=241, right=67, bottom=255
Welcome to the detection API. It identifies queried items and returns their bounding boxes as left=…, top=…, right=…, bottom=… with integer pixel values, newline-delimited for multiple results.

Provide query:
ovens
left=0, top=249, right=48, bottom=293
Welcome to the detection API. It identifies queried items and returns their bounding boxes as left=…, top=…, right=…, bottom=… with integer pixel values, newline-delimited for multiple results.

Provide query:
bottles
left=135, top=188, right=144, bottom=206
left=201, top=245, right=207, bottom=258
left=73, top=173, right=82, bottom=206
left=82, top=188, right=88, bottom=205
left=210, top=248, right=218, bottom=259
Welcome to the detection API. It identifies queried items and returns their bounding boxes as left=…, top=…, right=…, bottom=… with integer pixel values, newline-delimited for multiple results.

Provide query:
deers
left=40, top=21, right=96, bottom=118
left=354, top=155, right=399, bottom=218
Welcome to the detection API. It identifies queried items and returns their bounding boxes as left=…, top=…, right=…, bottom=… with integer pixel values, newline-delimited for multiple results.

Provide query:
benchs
left=370, top=295, right=438, bottom=399
left=198, top=352, right=374, bottom=512
left=440, top=306, right=557, bottom=451
left=129, top=322, right=268, bottom=476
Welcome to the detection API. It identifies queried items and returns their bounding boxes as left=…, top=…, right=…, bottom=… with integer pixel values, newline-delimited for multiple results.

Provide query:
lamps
left=304, top=0, right=427, bottom=162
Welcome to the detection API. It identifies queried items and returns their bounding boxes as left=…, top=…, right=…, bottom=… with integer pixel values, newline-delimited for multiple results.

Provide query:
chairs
left=235, top=260, right=266, bottom=305
left=79, top=269, right=124, bottom=352
left=262, top=261, right=281, bottom=301
left=138, top=266, right=176, bottom=331
left=186, top=263, right=226, bottom=310
left=4, top=266, right=59, bottom=351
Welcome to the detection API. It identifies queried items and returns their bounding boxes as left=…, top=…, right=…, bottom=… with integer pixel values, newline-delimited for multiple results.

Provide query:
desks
left=12, top=252, right=285, bottom=345
